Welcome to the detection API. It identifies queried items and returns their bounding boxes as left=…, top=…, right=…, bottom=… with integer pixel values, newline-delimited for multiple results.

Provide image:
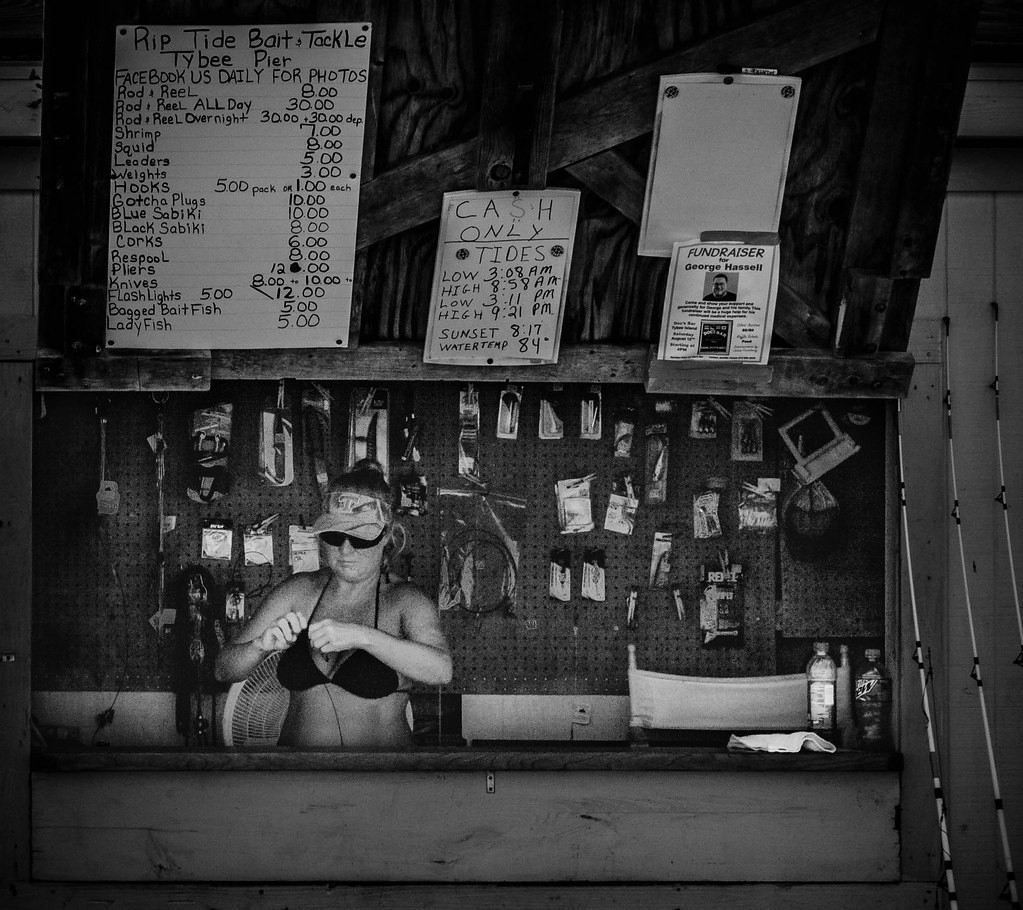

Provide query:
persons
left=702, top=275, right=737, bottom=303
left=212, top=460, right=454, bottom=749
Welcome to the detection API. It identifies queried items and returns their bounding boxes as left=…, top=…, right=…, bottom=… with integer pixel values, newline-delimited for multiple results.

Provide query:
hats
left=310, top=492, right=393, bottom=541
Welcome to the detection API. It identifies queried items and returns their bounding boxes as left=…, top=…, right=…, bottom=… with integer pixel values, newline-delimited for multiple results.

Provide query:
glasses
left=319, top=525, right=386, bottom=549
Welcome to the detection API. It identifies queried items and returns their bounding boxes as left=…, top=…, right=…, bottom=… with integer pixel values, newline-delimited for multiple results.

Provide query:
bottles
left=854, top=649, right=892, bottom=756
left=806, top=642, right=836, bottom=745
left=836, top=645, right=853, bottom=730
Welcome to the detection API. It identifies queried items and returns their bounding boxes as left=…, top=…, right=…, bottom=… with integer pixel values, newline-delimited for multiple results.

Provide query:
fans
left=224, top=645, right=412, bottom=748
